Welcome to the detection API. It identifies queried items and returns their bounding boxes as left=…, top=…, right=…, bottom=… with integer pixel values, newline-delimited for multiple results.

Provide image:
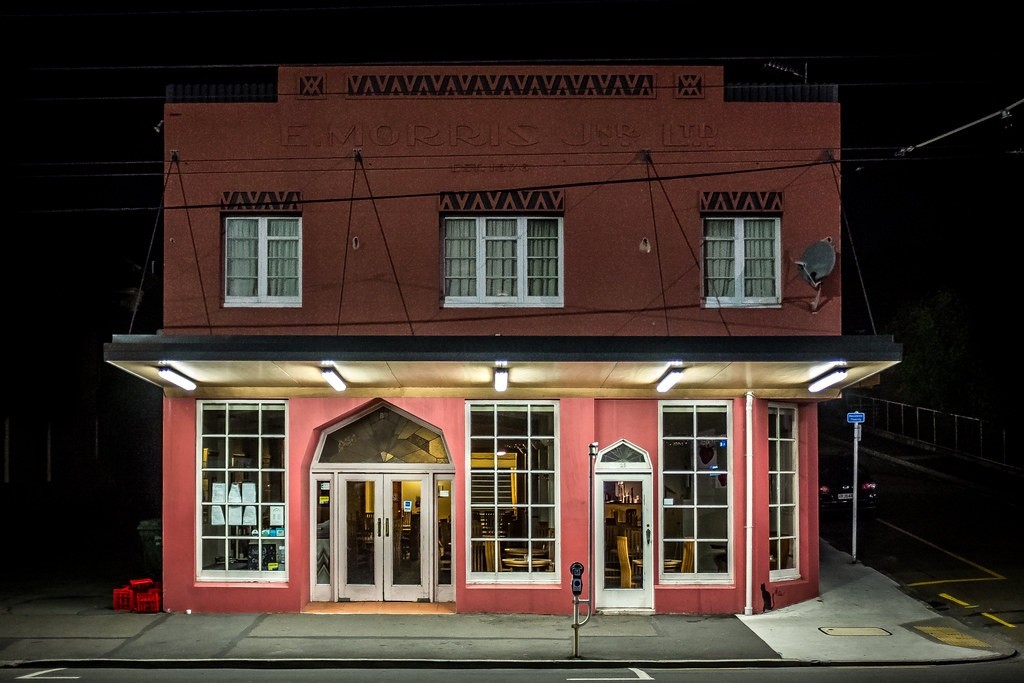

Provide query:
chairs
left=615, top=536, right=643, bottom=588
left=681, top=537, right=696, bottom=573
left=484, top=536, right=513, bottom=572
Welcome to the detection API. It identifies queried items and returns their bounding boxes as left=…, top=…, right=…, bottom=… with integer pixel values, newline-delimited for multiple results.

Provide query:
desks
left=632, top=559, right=682, bottom=569
left=501, top=547, right=551, bottom=572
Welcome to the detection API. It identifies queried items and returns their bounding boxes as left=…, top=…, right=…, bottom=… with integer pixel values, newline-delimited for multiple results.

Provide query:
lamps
left=807, top=368, right=848, bottom=393
left=321, top=367, right=347, bottom=392
left=494, top=367, right=509, bottom=393
left=158, top=368, right=197, bottom=391
left=656, top=367, right=683, bottom=393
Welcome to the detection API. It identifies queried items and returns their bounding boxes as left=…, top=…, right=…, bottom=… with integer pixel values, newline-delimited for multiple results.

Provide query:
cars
left=817, top=451, right=878, bottom=513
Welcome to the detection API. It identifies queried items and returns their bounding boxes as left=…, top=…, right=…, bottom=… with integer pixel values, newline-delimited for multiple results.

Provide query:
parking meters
left=571, top=561, right=590, bottom=656
